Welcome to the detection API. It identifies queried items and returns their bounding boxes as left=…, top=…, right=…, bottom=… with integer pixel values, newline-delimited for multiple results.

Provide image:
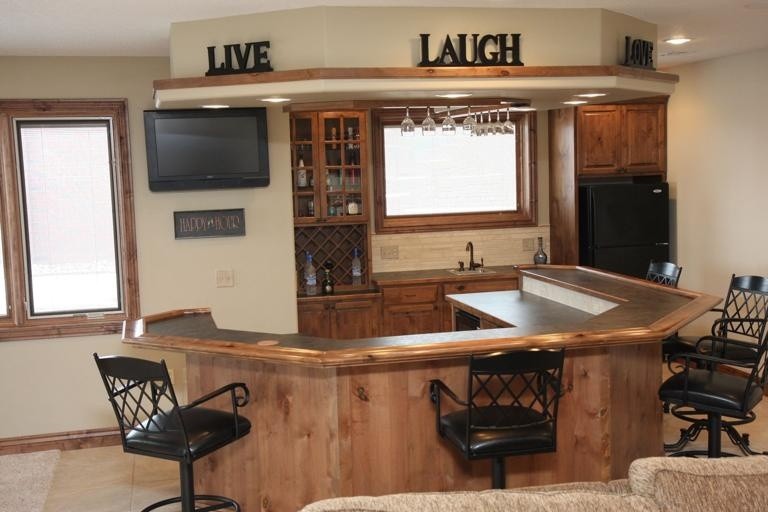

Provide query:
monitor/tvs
left=144, top=107, right=270, bottom=192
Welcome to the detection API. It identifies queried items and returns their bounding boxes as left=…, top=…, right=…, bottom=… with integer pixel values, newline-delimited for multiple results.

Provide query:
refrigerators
left=577, top=181, right=670, bottom=284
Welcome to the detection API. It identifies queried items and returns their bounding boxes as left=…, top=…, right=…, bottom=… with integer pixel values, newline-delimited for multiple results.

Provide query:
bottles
left=308, top=189, right=361, bottom=218
left=297, top=150, right=357, bottom=192
left=346, top=126, right=360, bottom=150
left=302, top=253, right=318, bottom=295
left=322, top=268, right=334, bottom=296
left=349, top=247, right=362, bottom=288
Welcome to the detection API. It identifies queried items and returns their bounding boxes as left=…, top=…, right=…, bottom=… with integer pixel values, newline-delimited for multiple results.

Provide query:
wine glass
left=395, top=105, right=515, bottom=138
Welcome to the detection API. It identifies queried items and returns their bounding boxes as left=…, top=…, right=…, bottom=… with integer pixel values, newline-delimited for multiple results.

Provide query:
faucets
left=466, top=241, right=484, bottom=270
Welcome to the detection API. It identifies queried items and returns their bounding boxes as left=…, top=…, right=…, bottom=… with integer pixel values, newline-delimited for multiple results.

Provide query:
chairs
left=645, top=260, right=682, bottom=289
left=664, top=271, right=768, bottom=372
left=654, top=326, right=768, bottom=459
left=91, top=350, right=254, bottom=511
left=424, top=342, right=571, bottom=493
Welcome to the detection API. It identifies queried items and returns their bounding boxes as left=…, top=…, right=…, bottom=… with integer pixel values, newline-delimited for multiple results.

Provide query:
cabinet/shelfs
left=442, top=278, right=519, bottom=335
left=287, top=107, right=378, bottom=229
left=545, top=94, right=669, bottom=179
left=295, top=289, right=382, bottom=341
left=377, top=281, right=444, bottom=339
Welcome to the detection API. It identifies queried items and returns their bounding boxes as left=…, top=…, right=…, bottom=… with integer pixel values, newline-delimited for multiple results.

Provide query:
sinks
left=447, top=267, right=498, bottom=275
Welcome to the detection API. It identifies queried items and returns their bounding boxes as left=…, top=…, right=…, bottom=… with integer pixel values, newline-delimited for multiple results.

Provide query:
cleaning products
left=322, top=260, right=334, bottom=294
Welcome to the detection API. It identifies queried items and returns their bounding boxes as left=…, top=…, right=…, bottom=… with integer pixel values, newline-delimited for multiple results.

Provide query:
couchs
left=298, top=453, right=767, bottom=512
